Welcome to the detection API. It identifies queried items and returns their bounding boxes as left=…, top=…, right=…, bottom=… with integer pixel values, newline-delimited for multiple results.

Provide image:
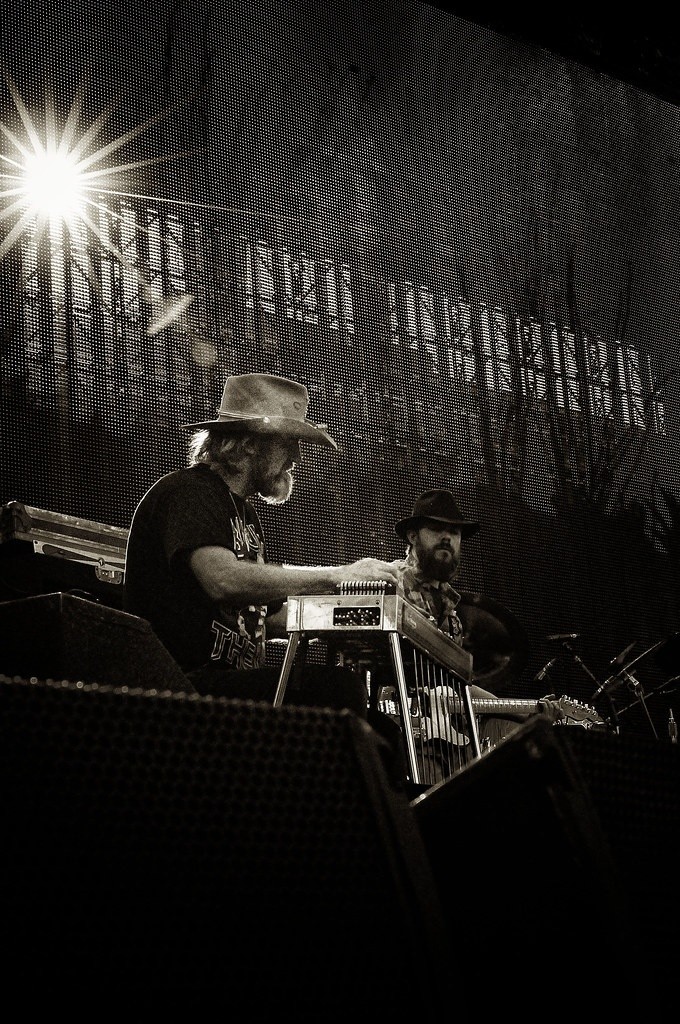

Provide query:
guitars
left=375, top=682, right=609, bottom=747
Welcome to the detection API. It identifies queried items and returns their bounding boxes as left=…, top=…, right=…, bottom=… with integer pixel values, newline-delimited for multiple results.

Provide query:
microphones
left=533, top=658, right=556, bottom=683
left=611, top=642, right=636, bottom=666
left=547, top=633, right=580, bottom=644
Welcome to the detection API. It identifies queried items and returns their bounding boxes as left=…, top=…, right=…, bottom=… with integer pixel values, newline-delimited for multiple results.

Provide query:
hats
left=180, top=372, right=338, bottom=452
left=394, top=489, right=480, bottom=545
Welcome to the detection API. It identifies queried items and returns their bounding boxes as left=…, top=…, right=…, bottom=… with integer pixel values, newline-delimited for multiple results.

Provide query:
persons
left=363, top=490, right=565, bottom=792
left=122, top=371, right=412, bottom=710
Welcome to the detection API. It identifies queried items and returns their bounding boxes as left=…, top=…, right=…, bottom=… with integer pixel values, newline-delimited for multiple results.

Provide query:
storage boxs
left=0, top=501, right=130, bottom=607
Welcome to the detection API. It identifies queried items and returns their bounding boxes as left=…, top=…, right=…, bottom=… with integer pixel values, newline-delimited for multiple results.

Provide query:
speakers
left=0, top=591, right=680, bottom=1024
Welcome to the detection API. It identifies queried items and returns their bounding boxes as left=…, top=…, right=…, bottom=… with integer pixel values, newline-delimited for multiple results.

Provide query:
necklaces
left=230, top=492, right=250, bottom=556
